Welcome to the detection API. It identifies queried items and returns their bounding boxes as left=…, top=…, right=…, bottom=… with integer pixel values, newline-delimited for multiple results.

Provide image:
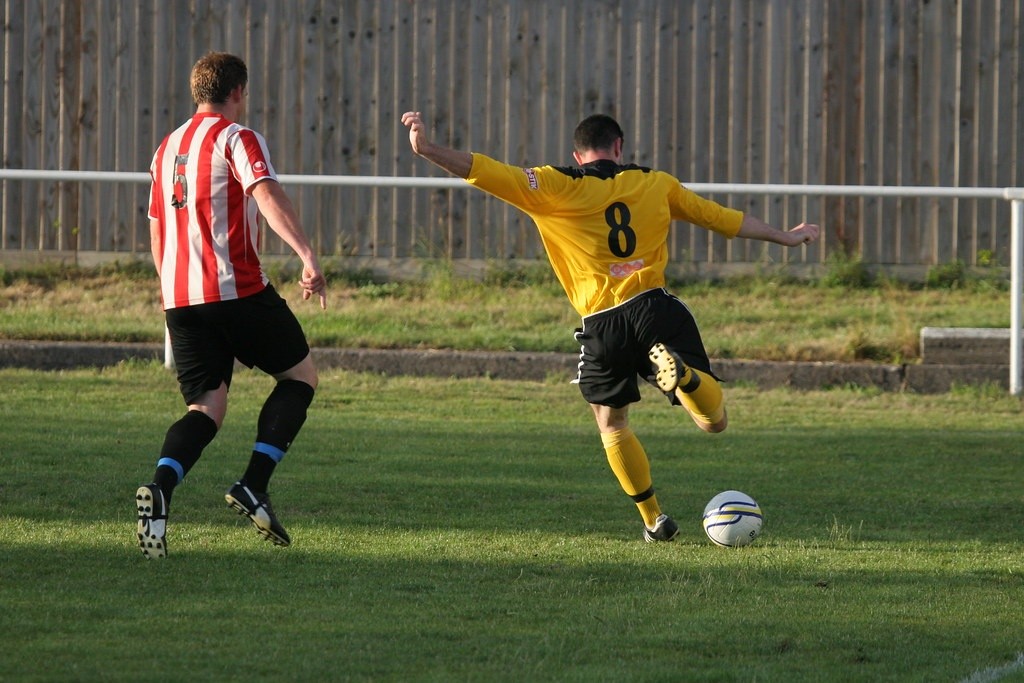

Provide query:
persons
left=401, top=111, right=819, bottom=541
left=136, top=52, right=327, bottom=558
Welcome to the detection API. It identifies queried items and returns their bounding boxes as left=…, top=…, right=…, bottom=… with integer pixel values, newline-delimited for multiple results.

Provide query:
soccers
left=703, top=489, right=764, bottom=548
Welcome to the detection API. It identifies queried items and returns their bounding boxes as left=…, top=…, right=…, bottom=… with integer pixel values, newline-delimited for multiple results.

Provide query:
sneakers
left=643, top=513, right=679, bottom=544
left=224, top=480, right=293, bottom=549
left=135, top=483, right=170, bottom=561
left=646, top=343, right=684, bottom=393
